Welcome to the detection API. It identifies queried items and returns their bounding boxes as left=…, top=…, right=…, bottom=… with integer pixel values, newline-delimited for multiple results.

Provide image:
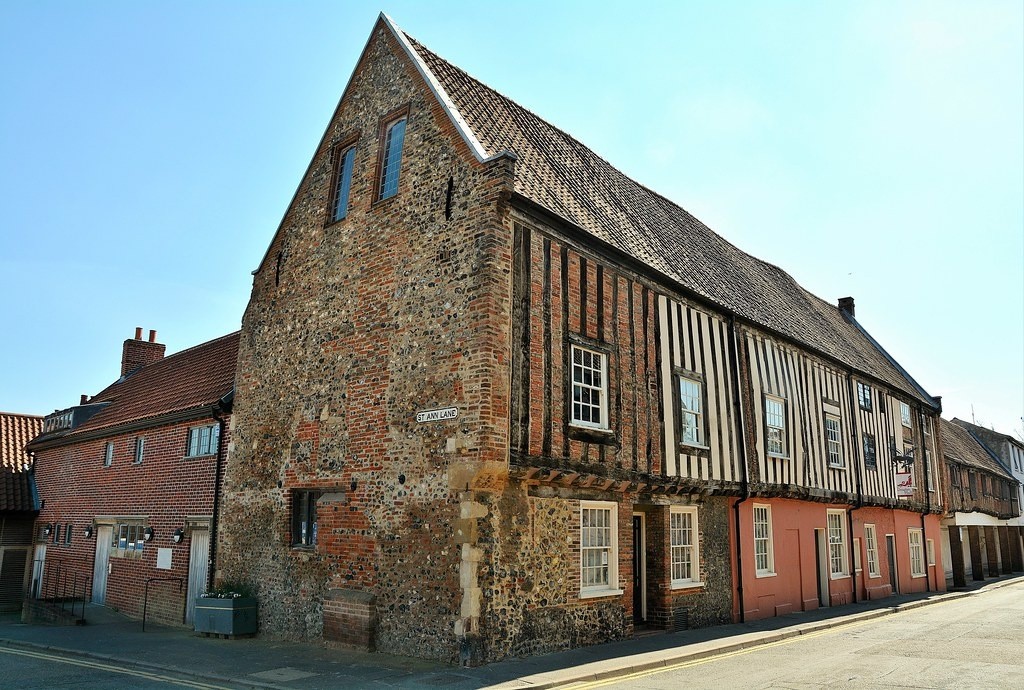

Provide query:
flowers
left=200, top=581, right=259, bottom=599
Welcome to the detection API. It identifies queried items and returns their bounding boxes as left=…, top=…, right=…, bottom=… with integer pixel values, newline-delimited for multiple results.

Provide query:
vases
left=194, top=596, right=256, bottom=634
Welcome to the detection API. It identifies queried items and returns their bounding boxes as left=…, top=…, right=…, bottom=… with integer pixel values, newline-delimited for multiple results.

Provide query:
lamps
left=174, top=527, right=184, bottom=542
left=84, top=526, right=93, bottom=538
left=45, top=521, right=53, bottom=536
left=144, top=527, right=154, bottom=541
